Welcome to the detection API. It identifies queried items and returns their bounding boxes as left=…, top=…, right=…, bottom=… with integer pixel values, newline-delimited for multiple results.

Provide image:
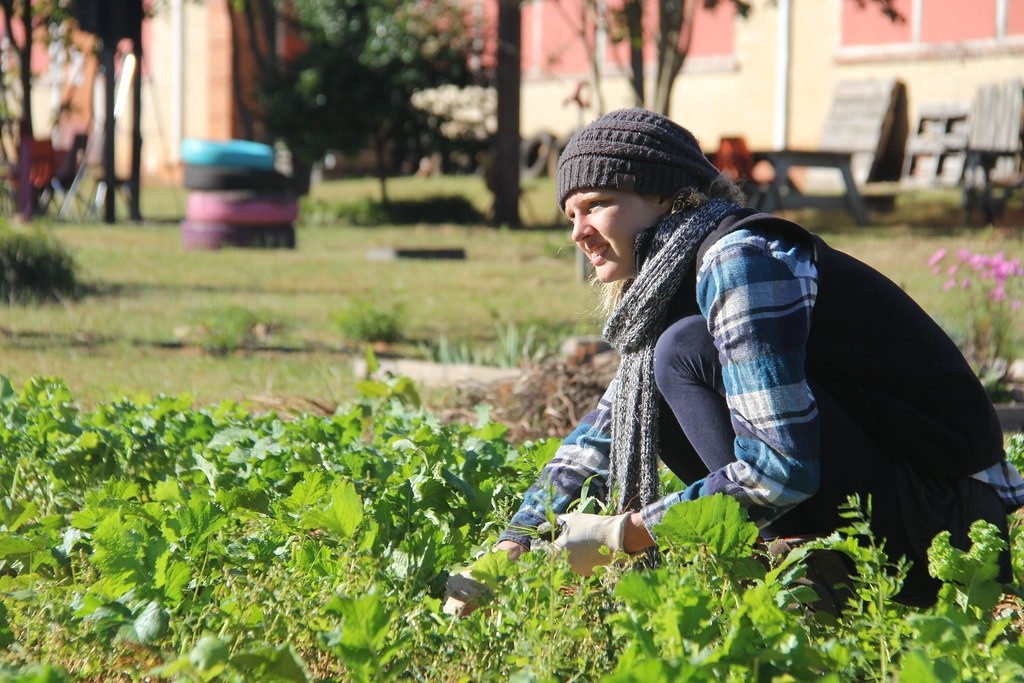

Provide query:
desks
left=83, top=177, right=132, bottom=221
left=706, top=149, right=870, bottom=226
left=947, top=145, right=1024, bottom=228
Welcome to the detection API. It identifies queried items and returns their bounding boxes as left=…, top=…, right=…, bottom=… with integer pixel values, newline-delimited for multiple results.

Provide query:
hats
left=556, top=107, right=718, bottom=211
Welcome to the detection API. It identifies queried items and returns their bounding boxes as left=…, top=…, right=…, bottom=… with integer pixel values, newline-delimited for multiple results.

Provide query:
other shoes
left=714, top=539, right=858, bottom=617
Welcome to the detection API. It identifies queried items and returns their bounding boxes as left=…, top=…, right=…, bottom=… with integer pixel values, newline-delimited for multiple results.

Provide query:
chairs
left=12, top=138, right=82, bottom=221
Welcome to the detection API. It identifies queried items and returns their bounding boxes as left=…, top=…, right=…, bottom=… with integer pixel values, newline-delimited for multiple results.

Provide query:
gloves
left=538, top=512, right=633, bottom=578
left=441, top=569, right=496, bottom=618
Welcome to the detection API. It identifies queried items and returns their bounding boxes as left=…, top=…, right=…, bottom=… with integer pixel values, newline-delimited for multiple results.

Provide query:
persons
left=438, top=107, right=1024, bottom=619
